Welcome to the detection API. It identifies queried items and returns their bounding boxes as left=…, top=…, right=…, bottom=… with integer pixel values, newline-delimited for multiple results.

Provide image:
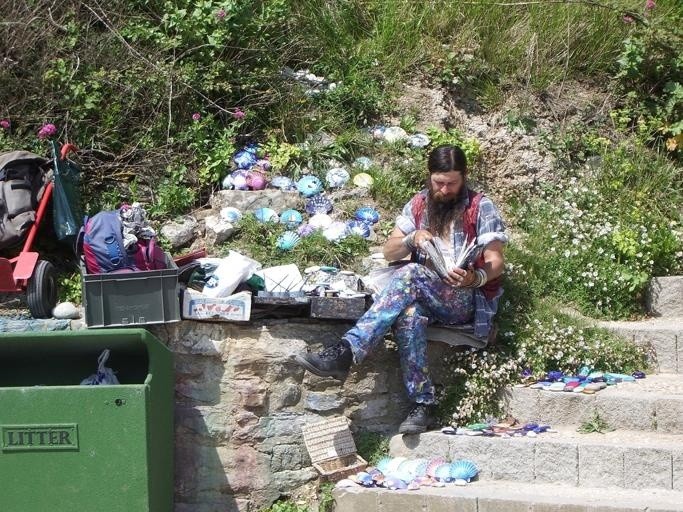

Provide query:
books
left=422, top=233, right=485, bottom=280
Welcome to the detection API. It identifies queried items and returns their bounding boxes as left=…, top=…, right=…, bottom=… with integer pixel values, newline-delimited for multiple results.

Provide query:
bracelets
left=401, top=229, right=417, bottom=252
left=466, top=266, right=488, bottom=288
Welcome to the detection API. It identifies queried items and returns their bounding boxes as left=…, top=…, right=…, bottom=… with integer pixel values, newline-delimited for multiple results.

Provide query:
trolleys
left=0, top=144, right=77, bottom=319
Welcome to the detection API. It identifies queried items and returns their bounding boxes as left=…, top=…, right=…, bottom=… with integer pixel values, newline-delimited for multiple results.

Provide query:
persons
left=294, top=144, right=511, bottom=435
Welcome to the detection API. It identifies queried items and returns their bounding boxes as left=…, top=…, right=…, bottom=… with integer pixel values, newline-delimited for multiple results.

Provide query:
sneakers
left=399, top=403, right=435, bottom=434
left=296, top=339, right=353, bottom=381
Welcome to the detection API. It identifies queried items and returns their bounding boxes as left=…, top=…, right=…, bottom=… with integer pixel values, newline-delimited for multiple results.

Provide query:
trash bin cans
left=0, top=328, right=175, bottom=512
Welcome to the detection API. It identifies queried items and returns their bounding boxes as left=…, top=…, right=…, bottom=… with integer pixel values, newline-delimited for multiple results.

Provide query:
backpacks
left=73, top=202, right=166, bottom=274
left=0, top=151, right=54, bottom=249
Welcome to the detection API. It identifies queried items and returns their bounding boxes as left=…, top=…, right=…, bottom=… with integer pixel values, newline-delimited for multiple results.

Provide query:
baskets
left=311, top=453, right=368, bottom=483
